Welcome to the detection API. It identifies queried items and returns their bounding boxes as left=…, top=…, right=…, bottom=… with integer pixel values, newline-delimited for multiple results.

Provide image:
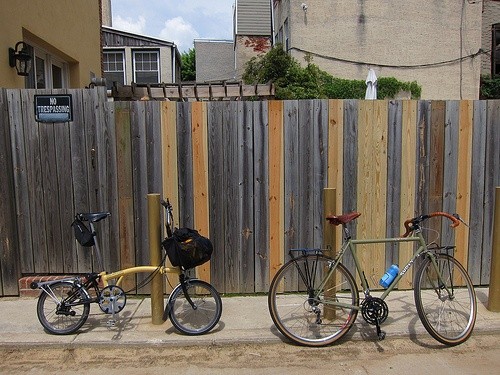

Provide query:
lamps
left=8, top=40, right=33, bottom=77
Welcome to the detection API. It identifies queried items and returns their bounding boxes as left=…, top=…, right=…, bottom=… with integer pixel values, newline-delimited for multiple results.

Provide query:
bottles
left=379, top=264, right=400, bottom=289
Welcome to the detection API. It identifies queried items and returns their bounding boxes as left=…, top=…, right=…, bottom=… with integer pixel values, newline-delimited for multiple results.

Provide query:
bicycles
left=267, top=211, right=478, bottom=347
left=30, top=197, right=223, bottom=336
